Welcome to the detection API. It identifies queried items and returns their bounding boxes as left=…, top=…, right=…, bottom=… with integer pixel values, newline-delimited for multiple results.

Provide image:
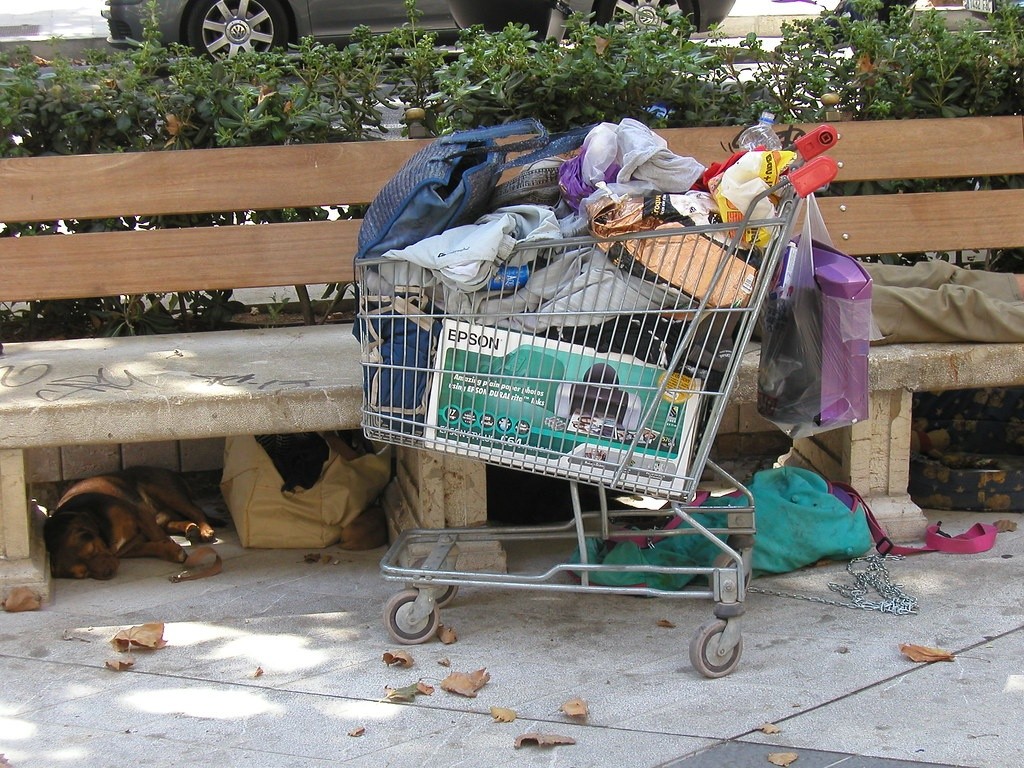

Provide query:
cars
left=104, top=1, right=733, bottom=70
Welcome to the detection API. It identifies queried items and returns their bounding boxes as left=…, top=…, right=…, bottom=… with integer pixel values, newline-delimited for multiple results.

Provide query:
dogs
left=43, top=465, right=229, bottom=580
left=338, top=506, right=388, bottom=550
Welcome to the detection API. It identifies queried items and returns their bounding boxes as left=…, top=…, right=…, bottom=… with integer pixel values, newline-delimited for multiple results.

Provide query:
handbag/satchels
left=220, top=431, right=392, bottom=548
left=358, top=117, right=603, bottom=260
left=756, top=192, right=872, bottom=438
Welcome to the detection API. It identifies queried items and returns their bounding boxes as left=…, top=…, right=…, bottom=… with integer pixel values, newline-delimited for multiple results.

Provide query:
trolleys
left=352, top=123, right=843, bottom=678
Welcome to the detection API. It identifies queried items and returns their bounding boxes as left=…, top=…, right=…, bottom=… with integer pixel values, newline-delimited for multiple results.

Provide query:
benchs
left=0, top=116, right=1024, bottom=613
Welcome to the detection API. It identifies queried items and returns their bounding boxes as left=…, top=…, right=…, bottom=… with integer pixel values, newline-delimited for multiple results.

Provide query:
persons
left=732, top=244, right=1024, bottom=348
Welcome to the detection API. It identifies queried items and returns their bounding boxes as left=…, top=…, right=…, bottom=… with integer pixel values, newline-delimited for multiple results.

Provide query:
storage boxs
left=423, top=318, right=699, bottom=497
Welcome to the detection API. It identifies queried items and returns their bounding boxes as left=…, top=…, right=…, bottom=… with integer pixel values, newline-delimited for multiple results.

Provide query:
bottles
left=478, top=262, right=551, bottom=291
left=739, top=112, right=783, bottom=151
